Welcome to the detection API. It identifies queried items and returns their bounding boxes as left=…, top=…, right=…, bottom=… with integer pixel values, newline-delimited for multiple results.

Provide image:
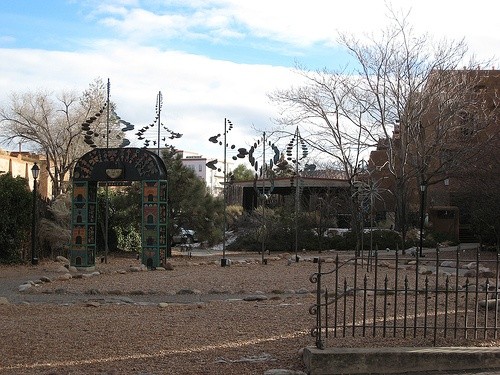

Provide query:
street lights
left=27, top=162, right=42, bottom=264
left=418, top=181, right=426, bottom=257
